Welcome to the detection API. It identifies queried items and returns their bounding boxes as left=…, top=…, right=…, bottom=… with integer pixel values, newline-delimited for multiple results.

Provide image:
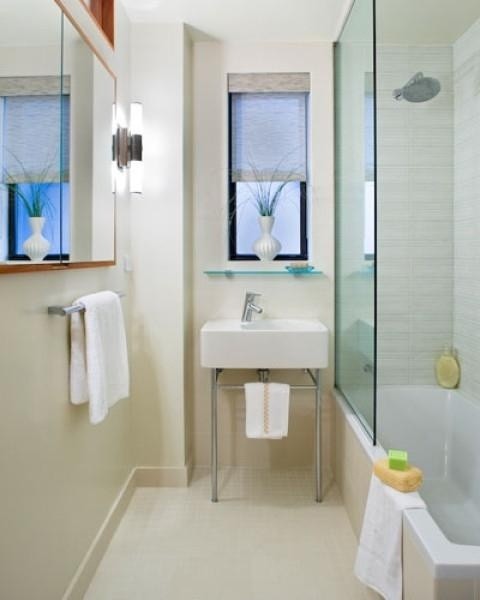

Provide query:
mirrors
left=2, top=0, right=117, bottom=271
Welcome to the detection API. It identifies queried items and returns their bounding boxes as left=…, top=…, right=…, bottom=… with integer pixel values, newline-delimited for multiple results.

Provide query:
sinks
left=199, top=320, right=328, bottom=370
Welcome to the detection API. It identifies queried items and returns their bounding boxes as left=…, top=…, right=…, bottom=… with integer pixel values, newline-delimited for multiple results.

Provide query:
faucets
left=240, top=291, right=264, bottom=321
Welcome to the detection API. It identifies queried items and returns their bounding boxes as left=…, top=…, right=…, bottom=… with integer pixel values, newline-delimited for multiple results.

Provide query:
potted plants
left=7, top=145, right=55, bottom=262
left=237, top=137, right=307, bottom=261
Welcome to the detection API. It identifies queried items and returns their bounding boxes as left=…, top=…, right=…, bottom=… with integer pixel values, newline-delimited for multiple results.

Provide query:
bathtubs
left=333, top=385, right=479, bottom=596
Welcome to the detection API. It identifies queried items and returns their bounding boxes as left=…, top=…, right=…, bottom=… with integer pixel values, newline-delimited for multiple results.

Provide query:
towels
left=243, top=381, right=289, bottom=441
left=352, top=471, right=426, bottom=600
left=68, top=290, right=129, bottom=425
